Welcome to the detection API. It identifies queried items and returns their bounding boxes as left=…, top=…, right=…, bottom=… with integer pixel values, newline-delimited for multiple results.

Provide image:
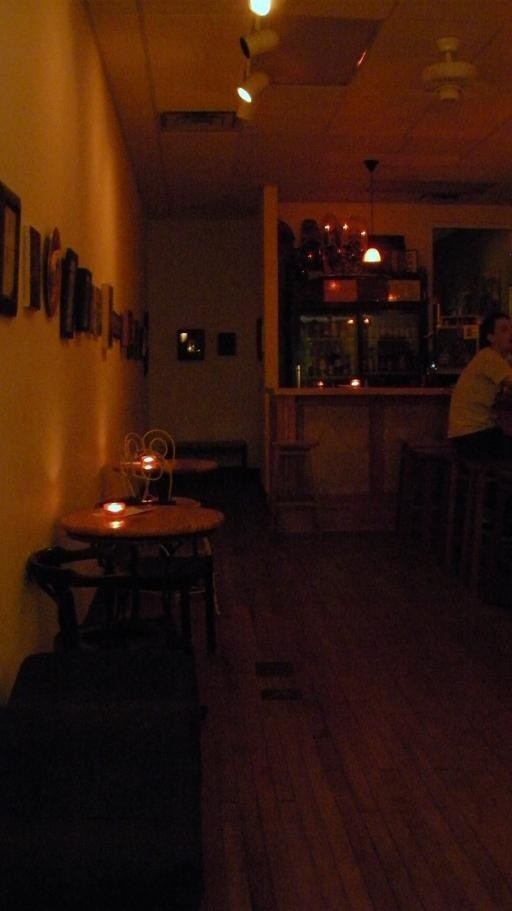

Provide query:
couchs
left=169, top=439, right=247, bottom=511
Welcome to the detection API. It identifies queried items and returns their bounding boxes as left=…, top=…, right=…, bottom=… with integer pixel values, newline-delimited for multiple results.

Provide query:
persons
left=447, top=312, right=512, bottom=573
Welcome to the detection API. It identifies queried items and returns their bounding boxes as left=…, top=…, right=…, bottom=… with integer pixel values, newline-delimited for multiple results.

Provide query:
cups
left=102, top=503, right=126, bottom=529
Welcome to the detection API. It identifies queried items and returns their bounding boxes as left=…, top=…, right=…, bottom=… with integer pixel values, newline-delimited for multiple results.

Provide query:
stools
left=398, top=438, right=511, bottom=609
left=3, top=643, right=197, bottom=906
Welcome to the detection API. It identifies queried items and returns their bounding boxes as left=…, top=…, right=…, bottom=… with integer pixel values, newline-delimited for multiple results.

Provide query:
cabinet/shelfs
left=257, top=383, right=511, bottom=538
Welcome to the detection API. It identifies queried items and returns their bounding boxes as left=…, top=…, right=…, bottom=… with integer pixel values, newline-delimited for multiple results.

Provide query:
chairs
left=26, top=544, right=182, bottom=661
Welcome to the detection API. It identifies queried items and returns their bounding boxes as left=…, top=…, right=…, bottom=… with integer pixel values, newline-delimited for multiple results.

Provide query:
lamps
left=237, top=69, right=272, bottom=103
left=359, top=156, right=383, bottom=267
left=238, top=31, right=283, bottom=60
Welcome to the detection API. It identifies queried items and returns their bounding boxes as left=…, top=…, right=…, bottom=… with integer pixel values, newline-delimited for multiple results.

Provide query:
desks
left=127, top=451, right=219, bottom=502
left=66, top=505, right=226, bottom=658
